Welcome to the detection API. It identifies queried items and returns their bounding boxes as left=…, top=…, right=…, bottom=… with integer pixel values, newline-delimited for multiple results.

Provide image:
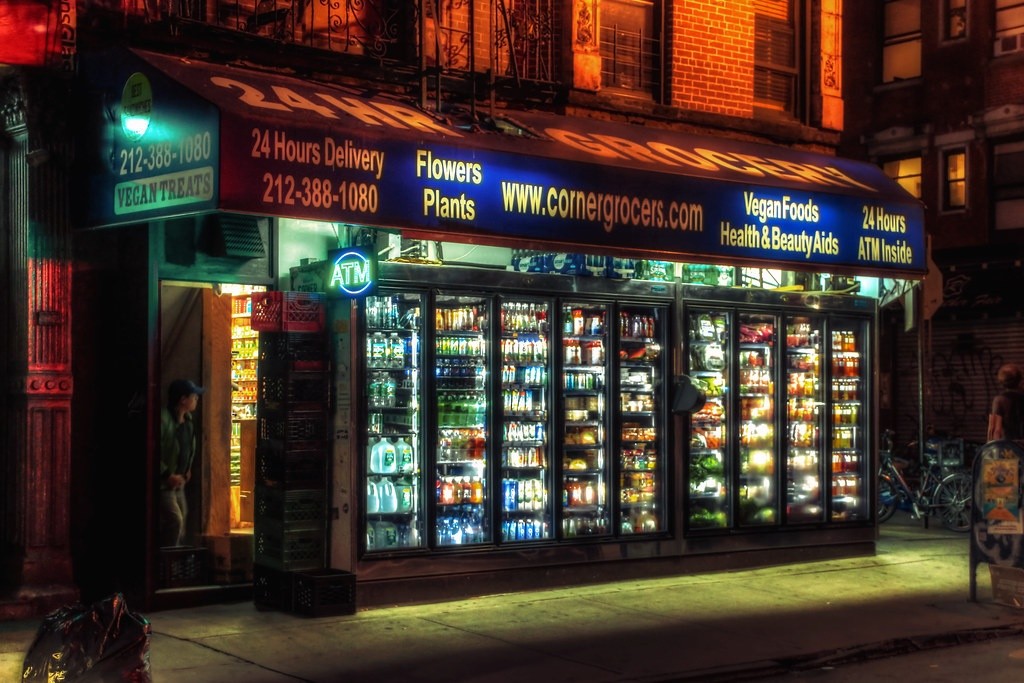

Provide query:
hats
left=998, top=364, right=1023, bottom=384
left=167, top=378, right=205, bottom=396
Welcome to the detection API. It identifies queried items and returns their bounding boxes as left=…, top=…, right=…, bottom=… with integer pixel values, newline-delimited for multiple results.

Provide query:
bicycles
left=876, top=428, right=972, bottom=534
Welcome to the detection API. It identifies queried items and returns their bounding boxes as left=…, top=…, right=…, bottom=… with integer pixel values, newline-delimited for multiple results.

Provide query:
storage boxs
left=202, top=533, right=252, bottom=585
left=158, top=544, right=209, bottom=588
left=989, top=565, right=1024, bottom=608
left=283, top=567, right=357, bottom=618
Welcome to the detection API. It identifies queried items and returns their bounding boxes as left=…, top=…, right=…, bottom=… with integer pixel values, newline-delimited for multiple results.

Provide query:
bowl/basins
left=622, top=393, right=653, bottom=412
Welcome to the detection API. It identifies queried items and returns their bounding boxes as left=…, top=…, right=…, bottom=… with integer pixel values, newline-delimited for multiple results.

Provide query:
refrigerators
left=290, top=259, right=677, bottom=579
left=670, top=282, right=880, bottom=554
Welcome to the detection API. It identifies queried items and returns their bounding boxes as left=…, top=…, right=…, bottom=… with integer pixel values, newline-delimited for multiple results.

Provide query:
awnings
left=80, top=48, right=930, bottom=307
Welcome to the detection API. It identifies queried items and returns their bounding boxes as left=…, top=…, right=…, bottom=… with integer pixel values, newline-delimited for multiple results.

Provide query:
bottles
left=831, top=331, right=862, bottom=496
left=621, top=509, right=655, bottom=533
left=619, top=310, right=655, bottom=338
left=436, top=305, right=488, bottom=333
left=503, top=387, right=532, bottom=411
left=562, top=477, right=595, bottom=508
left=562, top=511, right=607, bottom=537
left=436, top=505, right=484, bottom=544
left=435, top=429, right=484, bottom=504
left=787, top=354, right=818, bottom=397
left=436, top=358, right=485, bottom=425
left=369, top=372, right=397, bottom=408
left=501, top=336, right=547, bottom=385
left=500, top=302, right=547, bottom=332
left=517, top=519, right=533, bottom=541
left=399, top=395, right=420, bottom=432
left=366, top=294, right=420, bottom=330
left=365, top=332, right=405, bottom=369
left=367, top=436, right=414, bottom=549
left=503, top=420, right=545, bottom=466
left=563, top=306, right=605, bottom=390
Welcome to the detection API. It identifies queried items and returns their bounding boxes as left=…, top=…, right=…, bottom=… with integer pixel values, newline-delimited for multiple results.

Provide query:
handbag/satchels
left=22, top=591, right=152, bottom=683
left=987, top=414, right=1004, bottom=441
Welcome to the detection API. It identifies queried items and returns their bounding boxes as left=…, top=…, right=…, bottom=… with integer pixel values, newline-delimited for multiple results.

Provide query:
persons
left=986, top=362, right=1024, bottom=442
left=160, top=379, right=206, bottom=546
